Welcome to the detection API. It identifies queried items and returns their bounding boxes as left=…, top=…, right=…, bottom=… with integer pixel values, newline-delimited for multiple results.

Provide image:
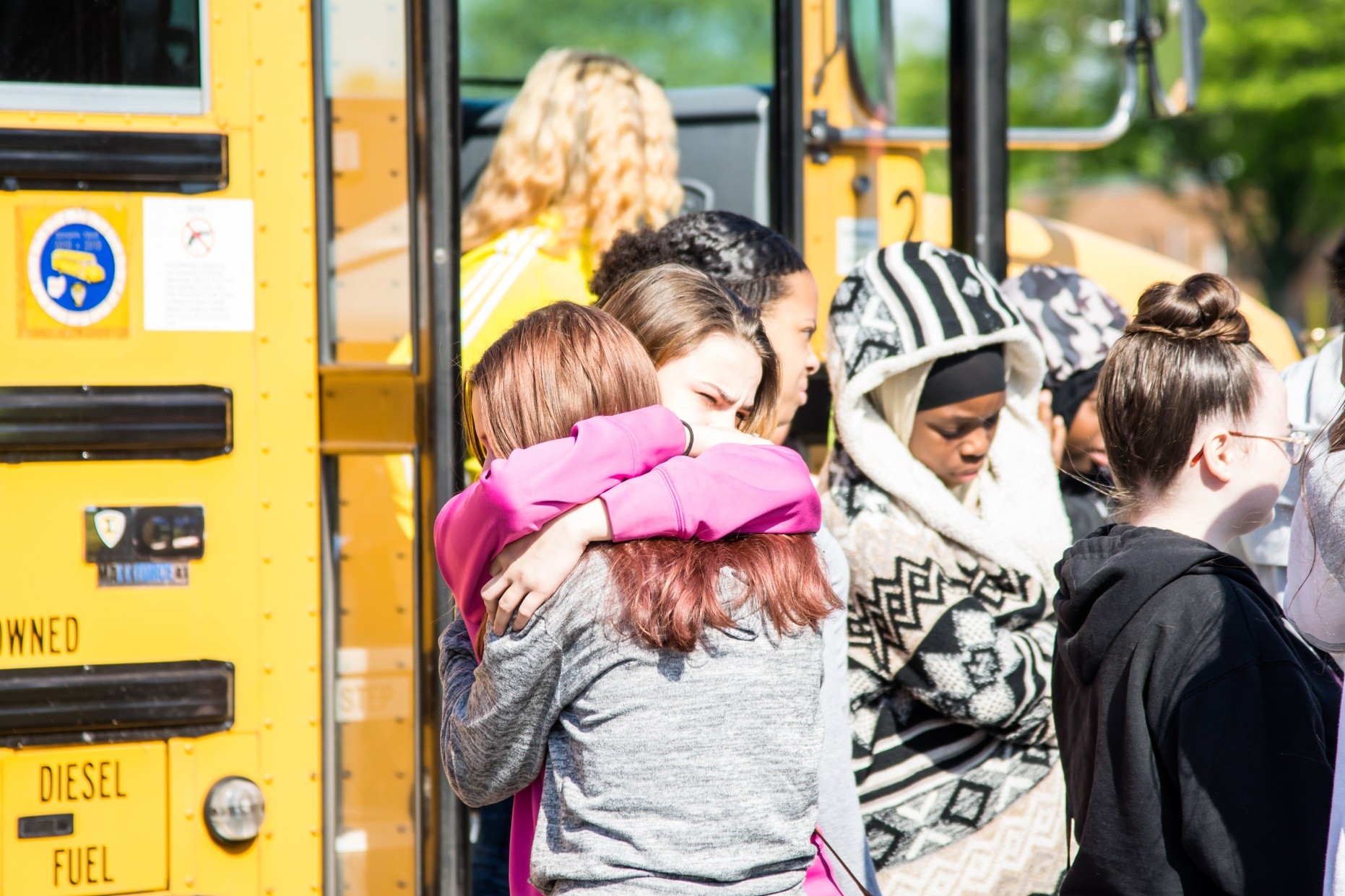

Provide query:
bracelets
left=679, top=419, right=694, bottom=455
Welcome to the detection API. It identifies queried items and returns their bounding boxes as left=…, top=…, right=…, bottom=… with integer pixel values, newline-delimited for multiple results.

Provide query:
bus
left=0, top=1, right=1208, bottom=896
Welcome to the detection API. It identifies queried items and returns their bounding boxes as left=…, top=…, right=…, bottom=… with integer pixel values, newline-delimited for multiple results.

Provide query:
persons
left=436, top=48, right=1345, bottom=896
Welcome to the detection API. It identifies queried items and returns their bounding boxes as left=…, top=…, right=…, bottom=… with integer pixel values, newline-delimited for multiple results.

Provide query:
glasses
left=1189, top=430, right=1311, bottom=468
